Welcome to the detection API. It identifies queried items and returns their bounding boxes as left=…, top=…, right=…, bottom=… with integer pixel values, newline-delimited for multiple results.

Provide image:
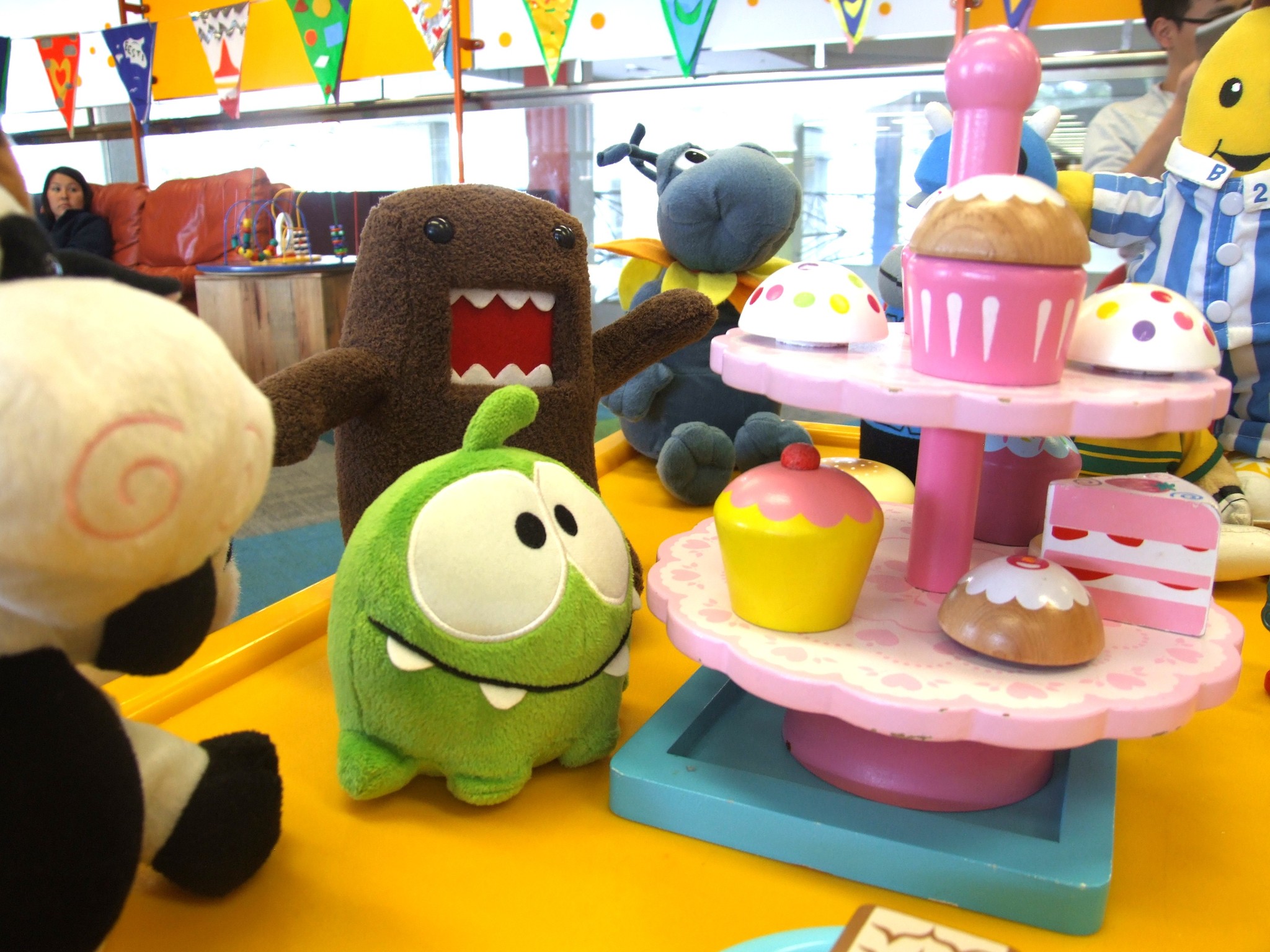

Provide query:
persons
left=40, top=166, right=115, bottom=260
left=1083, top=0, right=1250, bottom=269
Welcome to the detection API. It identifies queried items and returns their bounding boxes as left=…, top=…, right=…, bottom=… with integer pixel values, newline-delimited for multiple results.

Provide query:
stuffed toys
left=858, top=100, right=1061, bottom=479
left=256, top=184, right=718, bottom=548
left=326, top=385, right=642, bottom=807
left=1055, top=5, right=1270, bottom=459
left=1069, top=429, right=1251, bottom=526
left=0, top=189, right=281, bottom=952
left=597, top=122, right=813, bottom=507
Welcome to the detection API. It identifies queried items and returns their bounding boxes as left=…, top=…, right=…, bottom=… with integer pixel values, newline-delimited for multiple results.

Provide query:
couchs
left=85, top=167, right=295, bottom=317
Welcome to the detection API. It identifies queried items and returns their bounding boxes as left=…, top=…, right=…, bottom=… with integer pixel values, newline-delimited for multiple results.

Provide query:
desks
left=98, top=401, right=1270, bottom=952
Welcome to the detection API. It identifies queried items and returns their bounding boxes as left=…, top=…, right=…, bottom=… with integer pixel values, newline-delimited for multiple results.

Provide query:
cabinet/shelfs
left=610, top=329, right=1246, bottom=935
